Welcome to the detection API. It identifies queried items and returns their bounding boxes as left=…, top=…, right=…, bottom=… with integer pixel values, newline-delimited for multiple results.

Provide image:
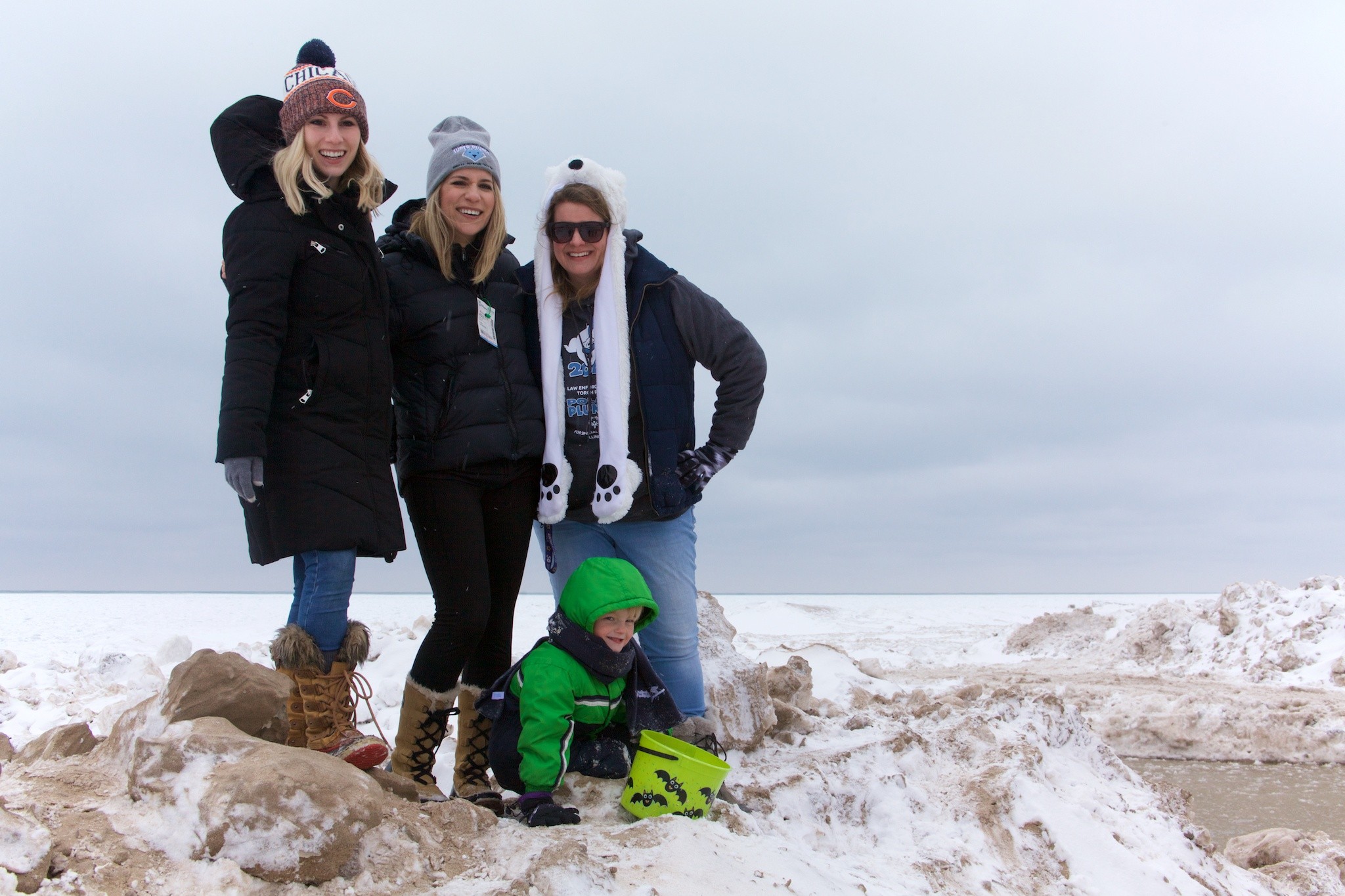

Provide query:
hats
left=424, top=116, right=501, bottom=200
left=532, top=156, right=642, bottom=526
left=279, top=38, right=368, bottom=145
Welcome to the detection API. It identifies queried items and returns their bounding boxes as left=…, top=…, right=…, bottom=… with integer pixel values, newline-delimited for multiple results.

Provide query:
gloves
left=223, top=457, right=263, bottom=503
left=675, top=440, right=738, bottom=494
left=518, top=792, right=581, bottom=828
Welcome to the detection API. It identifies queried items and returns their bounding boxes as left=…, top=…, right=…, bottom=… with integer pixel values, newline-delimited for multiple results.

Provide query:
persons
left=211, top=38, right=406, bottom=770
left=375, top=115, right=548, bottom=817
left=516, top=557, right=687, bottom=828
left=516, top=156, right=766, bottom=760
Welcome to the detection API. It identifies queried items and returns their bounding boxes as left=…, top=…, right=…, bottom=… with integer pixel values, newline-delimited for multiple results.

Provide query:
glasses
left=549, top=221, right=610, bottom=243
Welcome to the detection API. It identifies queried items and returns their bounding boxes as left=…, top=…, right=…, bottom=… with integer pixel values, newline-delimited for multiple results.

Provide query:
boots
left=277, top=617, right=392, bottom=771
left=270, top=638, right=307, bottom=749
left=448, top=684, right=502, bottom=803
left=670, top=705, right=749, bottom=812
left=383, top=670, right=461, bottom=802
